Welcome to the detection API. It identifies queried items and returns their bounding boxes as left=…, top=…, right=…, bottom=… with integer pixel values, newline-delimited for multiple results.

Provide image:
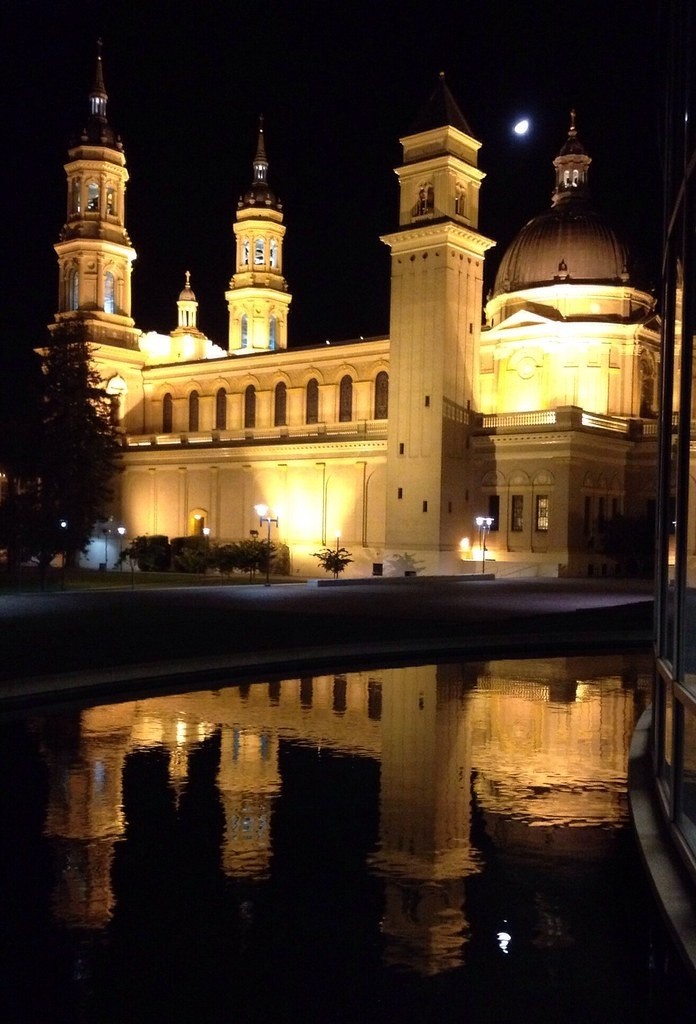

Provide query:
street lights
left=476, top=515, right=494, bottom=574
left=252, top=504, right=283, bottom=588
left=117, top=522, right=126, bottom=572
left=335, top=529, right=341, bottom=579
left=202, top=527, right=210, bottom=576
left=58, top=520, right=68, bottom=591
